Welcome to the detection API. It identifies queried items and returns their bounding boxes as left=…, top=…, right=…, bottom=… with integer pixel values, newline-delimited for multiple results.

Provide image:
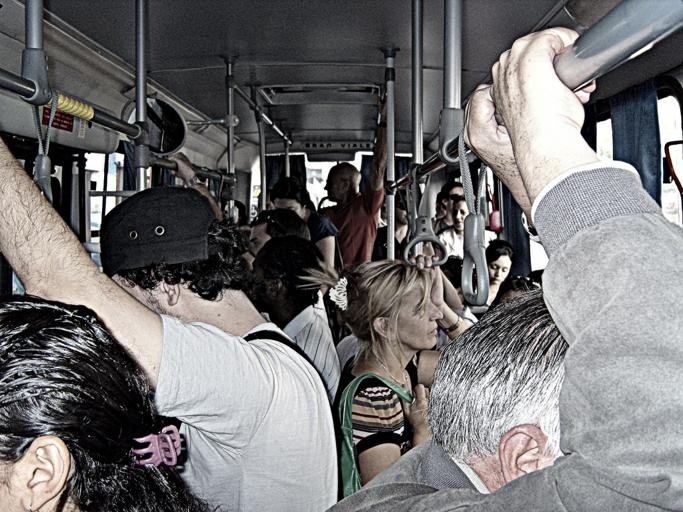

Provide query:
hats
left=99, top=184, right=220, bottom=276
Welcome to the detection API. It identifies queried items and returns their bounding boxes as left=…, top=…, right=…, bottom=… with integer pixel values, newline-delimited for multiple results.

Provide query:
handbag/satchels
left=337, top=370, right=413, bottom=501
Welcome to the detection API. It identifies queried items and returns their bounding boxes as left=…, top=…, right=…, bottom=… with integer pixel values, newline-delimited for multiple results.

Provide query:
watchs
left=380, top=117, right=386, bottom=127
left=440, top=316, right=462, bottom=335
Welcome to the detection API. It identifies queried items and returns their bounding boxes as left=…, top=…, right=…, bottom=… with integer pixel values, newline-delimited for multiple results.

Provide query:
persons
left=372, top=186, right=422, bottom=262
left=297, top=255, right=471, bottom=498
left=476, top=240, right=520, bottom=310
left=0, top=295, right=224, bottom=512
left=257, top=190, right=274, bottom=213
left=319, top=102, right=387, bottom=267
left=168, top=152, right=312, bottom=272
left=439, top=195, right=497, bottom=259
left=434, top=182, right=464, bottom=235
left=271, top=176, right=339, bottom=269
left=0, top=135, right=337, bottom=512
left=326, top=25, right=682, bottom=511
left=248, top=236, right=341, bottom=405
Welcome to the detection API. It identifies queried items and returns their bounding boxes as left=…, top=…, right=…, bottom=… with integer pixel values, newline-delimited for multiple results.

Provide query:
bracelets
left=185, top=176, right=199, bottom=187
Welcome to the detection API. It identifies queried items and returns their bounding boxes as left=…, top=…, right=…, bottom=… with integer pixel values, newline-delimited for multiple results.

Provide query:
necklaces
left=375, top=357, right=409, bottom=388
